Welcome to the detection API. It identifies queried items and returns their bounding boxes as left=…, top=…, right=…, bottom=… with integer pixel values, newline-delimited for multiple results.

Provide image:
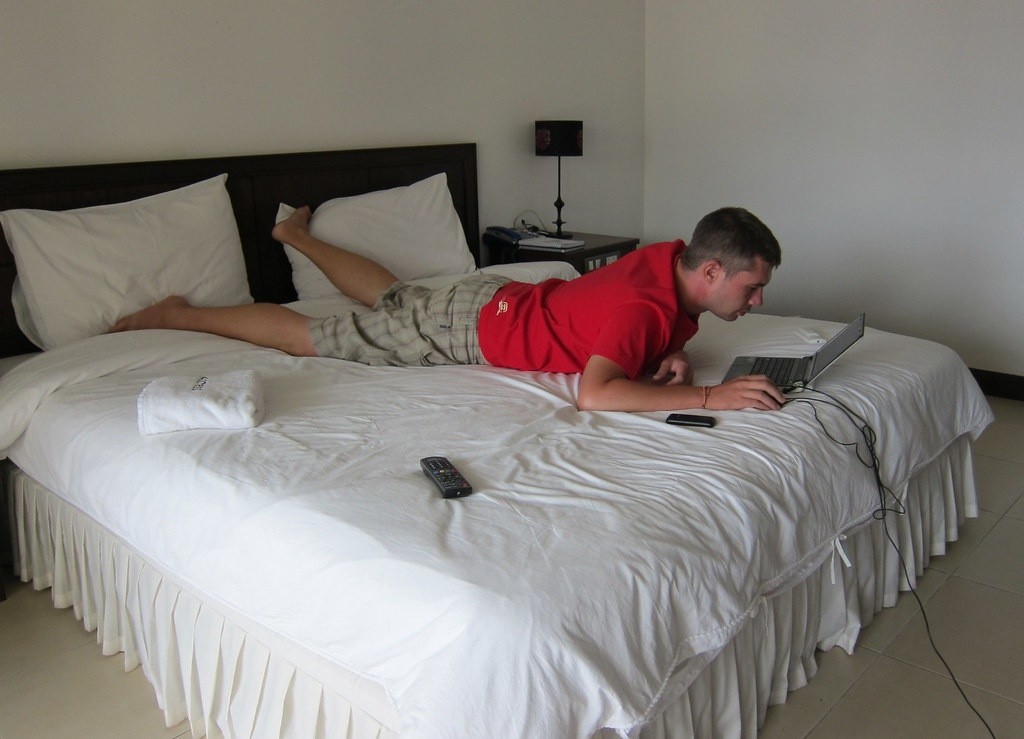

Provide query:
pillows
left=1, top=174, right=256, bottom=354
left=275, top=172, right=479, bottom=305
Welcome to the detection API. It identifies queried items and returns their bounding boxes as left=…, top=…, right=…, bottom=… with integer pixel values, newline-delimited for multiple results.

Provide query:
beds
left=1, top=142, right=995, bottom=739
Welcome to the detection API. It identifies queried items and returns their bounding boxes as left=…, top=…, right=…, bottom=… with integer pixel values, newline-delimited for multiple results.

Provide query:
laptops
left=722, top=313, right=865, bottom=392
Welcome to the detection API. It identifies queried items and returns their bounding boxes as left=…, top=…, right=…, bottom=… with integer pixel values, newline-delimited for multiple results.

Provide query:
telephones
left=487, top=226, right=521, bottom=242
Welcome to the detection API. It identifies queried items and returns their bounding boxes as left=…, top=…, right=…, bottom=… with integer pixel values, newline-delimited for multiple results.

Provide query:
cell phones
left=666, top=414, right=716, bottom=428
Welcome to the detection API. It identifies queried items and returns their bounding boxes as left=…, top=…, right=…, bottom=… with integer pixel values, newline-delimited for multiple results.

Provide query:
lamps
left=534, top=119, right=584, bottom=241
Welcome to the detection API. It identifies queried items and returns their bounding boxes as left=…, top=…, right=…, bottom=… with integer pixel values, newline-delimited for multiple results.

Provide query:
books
left=518, top=236, right=585, bottom=249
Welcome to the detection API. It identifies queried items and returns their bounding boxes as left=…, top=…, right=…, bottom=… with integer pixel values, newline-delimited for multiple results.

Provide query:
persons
left=105, top=204, right=788, bottom=414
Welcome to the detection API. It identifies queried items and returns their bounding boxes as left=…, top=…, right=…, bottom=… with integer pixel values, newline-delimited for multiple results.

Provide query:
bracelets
left=700, top=384, right=711, bottom=409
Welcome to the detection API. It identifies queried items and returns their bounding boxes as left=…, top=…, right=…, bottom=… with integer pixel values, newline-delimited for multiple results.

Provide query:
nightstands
left=487, top=231, right=641, bottom=275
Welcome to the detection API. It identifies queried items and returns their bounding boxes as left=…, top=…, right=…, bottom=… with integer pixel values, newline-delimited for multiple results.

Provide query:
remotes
left=420, top=457, right=472, bottom=498
